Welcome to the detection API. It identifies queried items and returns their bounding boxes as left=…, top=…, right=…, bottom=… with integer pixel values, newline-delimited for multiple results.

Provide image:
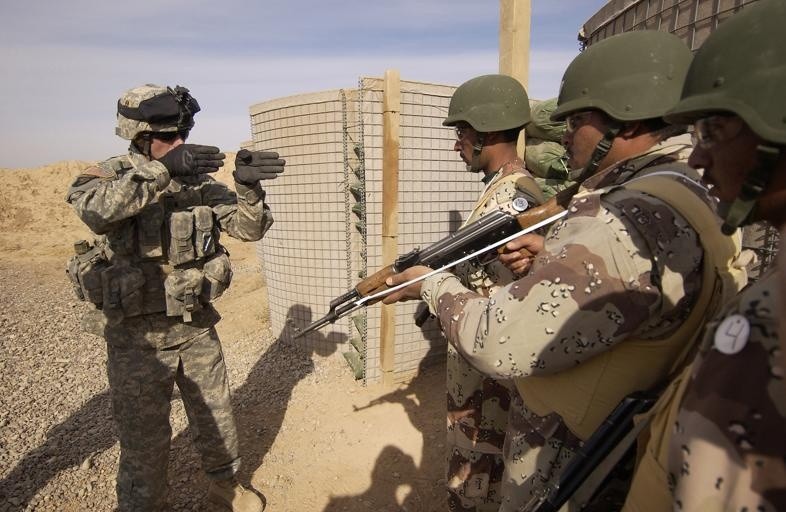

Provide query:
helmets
left=116, top=83, right=200, bottom=140
left=550, top=0, right=786, bottom=144
left=442, top=75, right=531, bottom=132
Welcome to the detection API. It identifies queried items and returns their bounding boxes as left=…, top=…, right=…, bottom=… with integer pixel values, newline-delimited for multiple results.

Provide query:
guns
left=522, top=391, right=659, bottom=511
left=292, top=182, right=579, bottom=339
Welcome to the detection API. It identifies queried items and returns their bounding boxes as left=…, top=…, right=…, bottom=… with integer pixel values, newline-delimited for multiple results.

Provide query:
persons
left=382, top=30, right=745, bottom=512
left=653, top=0, right=786, bottom=512
left=64, top=84, right=286, bottom=512
left=440, top=74, right=549, bottom=512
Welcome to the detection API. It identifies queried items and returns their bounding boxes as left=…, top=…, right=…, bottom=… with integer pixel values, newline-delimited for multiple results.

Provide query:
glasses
left=152, top=130, right=188, bottom=143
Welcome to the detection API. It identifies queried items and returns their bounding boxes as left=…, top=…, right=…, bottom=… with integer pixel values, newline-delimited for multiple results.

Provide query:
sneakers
left=209, top=479, right=263, bottom=512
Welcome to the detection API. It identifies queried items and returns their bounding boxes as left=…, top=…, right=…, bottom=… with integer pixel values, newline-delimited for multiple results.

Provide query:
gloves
left=158, top=144, right=226, bottom=177
left=235, top=149, right=285, bottom=184
